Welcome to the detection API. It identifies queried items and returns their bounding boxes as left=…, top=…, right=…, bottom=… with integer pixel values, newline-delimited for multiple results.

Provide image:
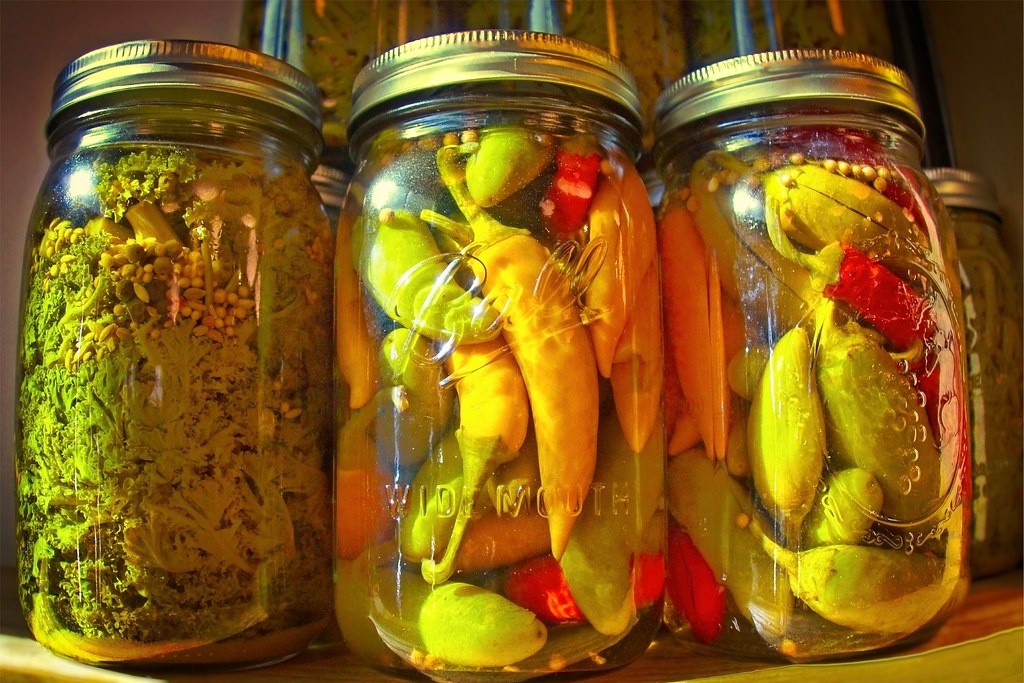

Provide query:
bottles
left=322, top=20, right=670, bottom=682
left=13, top=34, right=337, bottom=676
left=920, top=162, right=1022, bottom=583
left=633, top=45, right=975, bottom=668
left=232, top=0, right=920, bottom=190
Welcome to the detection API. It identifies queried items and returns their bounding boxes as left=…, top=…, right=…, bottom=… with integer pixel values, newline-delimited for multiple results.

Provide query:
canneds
left=15, top=30, right=1024, bottom=681
